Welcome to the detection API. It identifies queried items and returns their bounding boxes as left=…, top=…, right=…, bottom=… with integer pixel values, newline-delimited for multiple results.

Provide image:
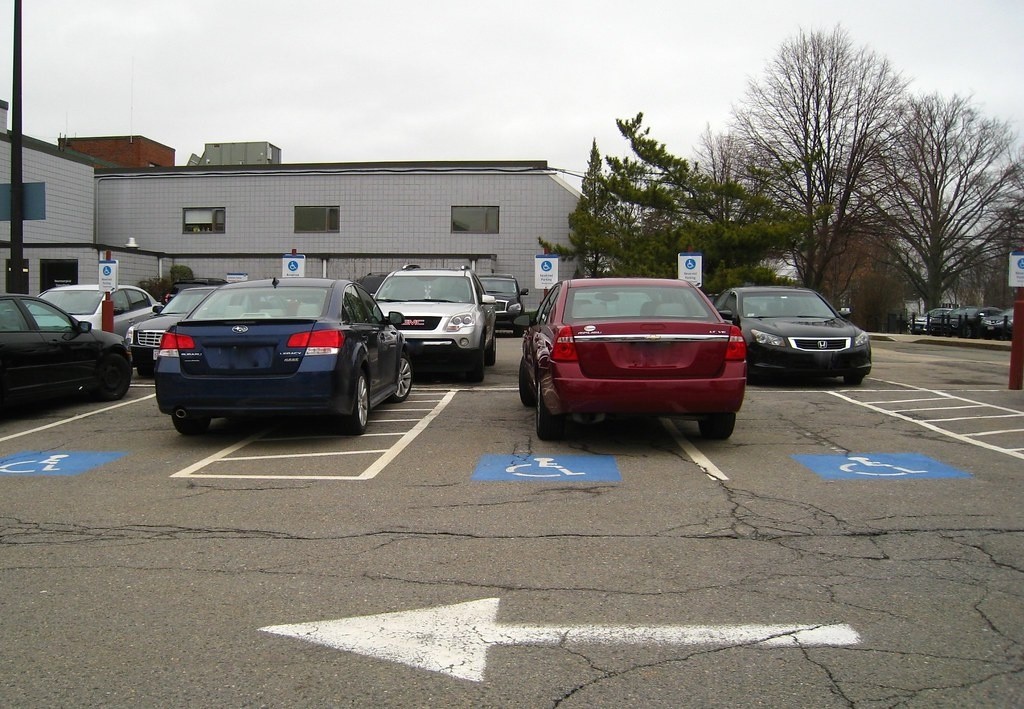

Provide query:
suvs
left=477, top=273, right=529, bottom=338
left=370, top=265, right=496, bottom=382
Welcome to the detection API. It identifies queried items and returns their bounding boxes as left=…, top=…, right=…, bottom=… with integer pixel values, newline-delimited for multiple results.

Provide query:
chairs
left=578, top=302, right=608, bottom=317
left=654, top=303, right=687, bottom=315
left=640, top=302, right=660, bottom=316
left=222, top=305, right=245, bottom=318
left=259, top=309, right=285, bottom=317
left=571, top=299, right=592, bottom=318
left=296, top=303, right=321, bottom=317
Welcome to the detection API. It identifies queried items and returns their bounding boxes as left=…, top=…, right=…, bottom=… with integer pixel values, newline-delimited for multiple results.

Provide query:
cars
left=125, top=287, right=218, bottom=377
left=21, top=284, right=165, bottom=336
left=0, top=293, right=132, bottom=402
left=929, top=306, right=1004, bottom=338
left=981, top=307, right=1015, bottom=341
left=908, top=308, right=953, bottom=334
left=153, top=276, right=415, bottom=436
left=714, top=286, right=872, bottom=386
left=519, top=279, right=747, bottom=440
